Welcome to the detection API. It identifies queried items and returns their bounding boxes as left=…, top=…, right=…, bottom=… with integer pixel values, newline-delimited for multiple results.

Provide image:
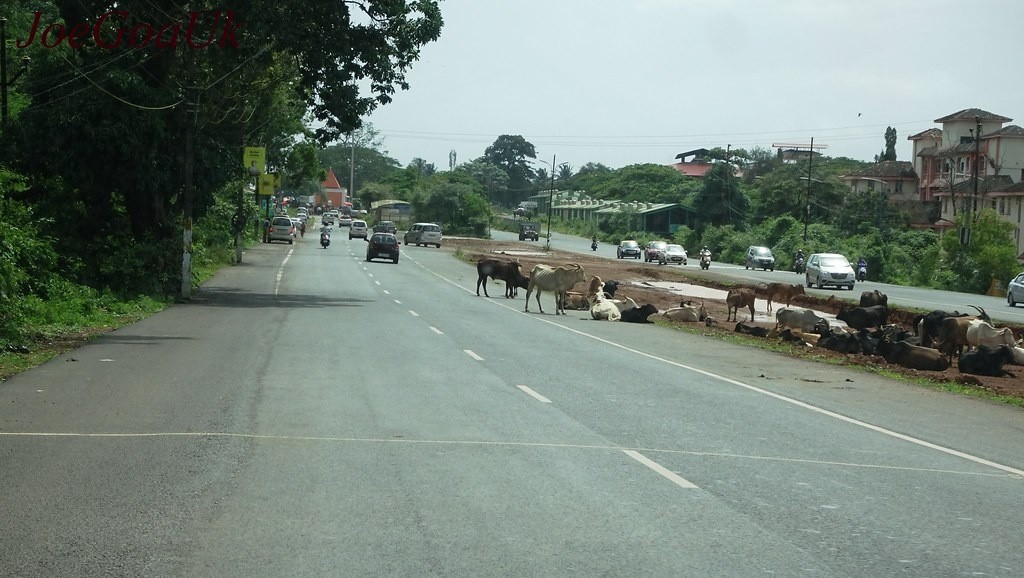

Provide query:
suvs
left=404, top=222, right=442, bottom=248
left=745, top=245, right=776, bottom=271
left=519, top=224, right=539, bottom=241
left=365, top=233, right=401, bottom=263
left=806, top=252, right=856, bottom=291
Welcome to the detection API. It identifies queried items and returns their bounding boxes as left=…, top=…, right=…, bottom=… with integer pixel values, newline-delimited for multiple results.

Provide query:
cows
left=475, top=253, right=1024, bottom=379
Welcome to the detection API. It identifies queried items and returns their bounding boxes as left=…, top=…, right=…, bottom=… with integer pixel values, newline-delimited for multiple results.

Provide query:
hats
left=798, top=250, right=802, bottom=252
left=704, top=246, right=707, bottom=248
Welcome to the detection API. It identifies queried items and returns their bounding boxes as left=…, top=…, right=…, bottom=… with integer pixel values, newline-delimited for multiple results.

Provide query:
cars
left=645, top=241, right=666, bottom=263
left=658, top=245, right=688, bottom=265
left=292, top=206, right=310, bottom=236
left=1006, top=272, right=1024, bottom=306
left=321, top=210, right=339, bottom=225
left=617, top=241, right=642, bottom=260
left=372, top=220, right=398, bottom=233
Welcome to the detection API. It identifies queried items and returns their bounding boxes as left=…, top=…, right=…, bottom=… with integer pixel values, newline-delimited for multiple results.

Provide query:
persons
left=592, top=235, right=597, bottom=244
left=299, top=221, right=306, bottom=237
left=320, top=221, right=331, bottom=241
left=699, top=245, right=712, bottom=263
left=795, top=249, right=805, bottom=266
left=857, top=256, right=868, bottom=273
left=261, top=218, right=271, bottom=243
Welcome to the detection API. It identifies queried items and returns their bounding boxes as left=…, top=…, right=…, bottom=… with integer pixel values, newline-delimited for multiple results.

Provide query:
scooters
left=793, top=257, right=805, bottom=275
left=700, top=254, right=712, bottom=270
left=591, top=241, right=598, bottom=251
left=857, top=264, right=867, bottom=282
left=320, top=227, right=333, bottom=249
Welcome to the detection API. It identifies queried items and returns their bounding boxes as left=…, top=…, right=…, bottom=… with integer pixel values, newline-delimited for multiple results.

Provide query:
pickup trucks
left=339, top=215, right=352, bottom=227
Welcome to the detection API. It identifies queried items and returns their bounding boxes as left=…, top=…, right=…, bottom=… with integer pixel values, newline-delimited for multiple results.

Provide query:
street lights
left=350, top=127, right=380, bottom=209
left=969, top=114, right=982, bottom=224
left=540, top=155, right=569, bottom=251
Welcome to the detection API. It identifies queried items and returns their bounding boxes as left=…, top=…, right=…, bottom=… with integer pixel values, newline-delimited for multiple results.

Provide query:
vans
left=267, top=216, right=294, bottom=244
left=348, top=221, right=368, bottom=241
